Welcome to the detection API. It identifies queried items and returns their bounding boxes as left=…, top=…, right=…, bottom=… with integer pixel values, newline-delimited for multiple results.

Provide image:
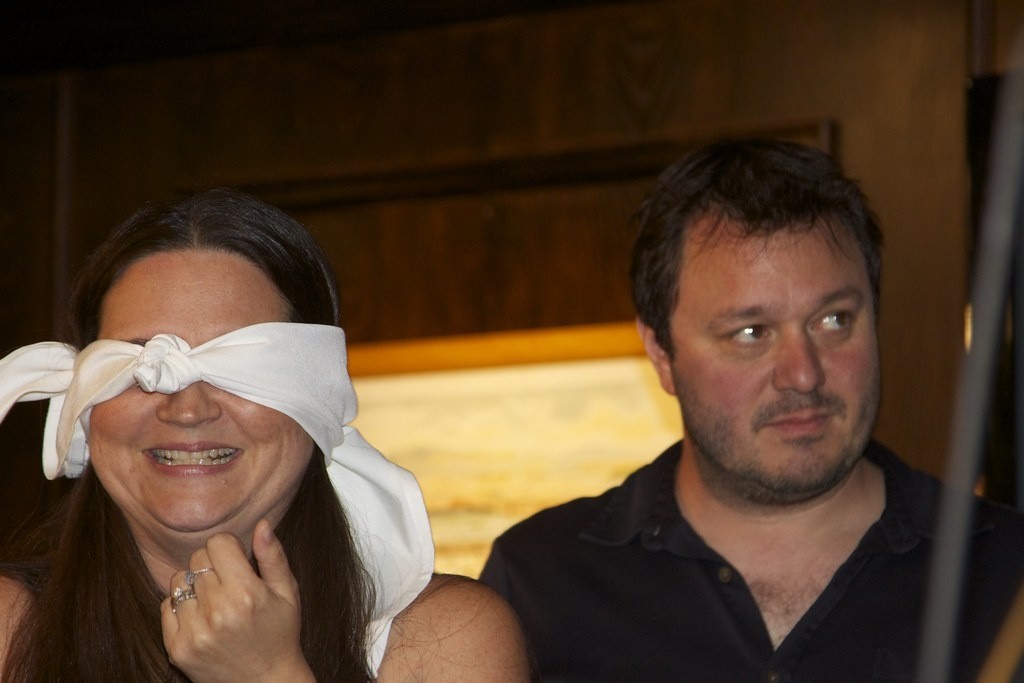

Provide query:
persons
left=0, top=189, right=537, bottom=683
left=478, top=133, right=1024, bottom=682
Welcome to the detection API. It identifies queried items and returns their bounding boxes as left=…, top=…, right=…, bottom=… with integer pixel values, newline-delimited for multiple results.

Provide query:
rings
left=170, top=567, right=213, bottom=614
